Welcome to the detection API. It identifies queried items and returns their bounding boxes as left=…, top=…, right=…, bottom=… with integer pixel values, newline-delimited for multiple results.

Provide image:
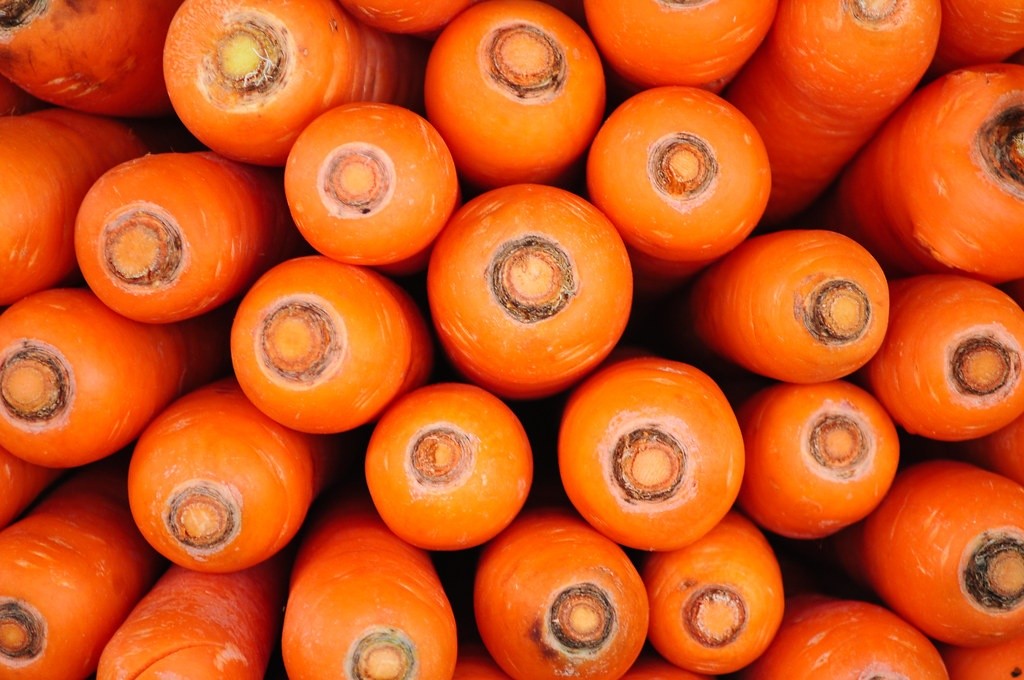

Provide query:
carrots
left=0, top=0, right=1024, bottom=680
